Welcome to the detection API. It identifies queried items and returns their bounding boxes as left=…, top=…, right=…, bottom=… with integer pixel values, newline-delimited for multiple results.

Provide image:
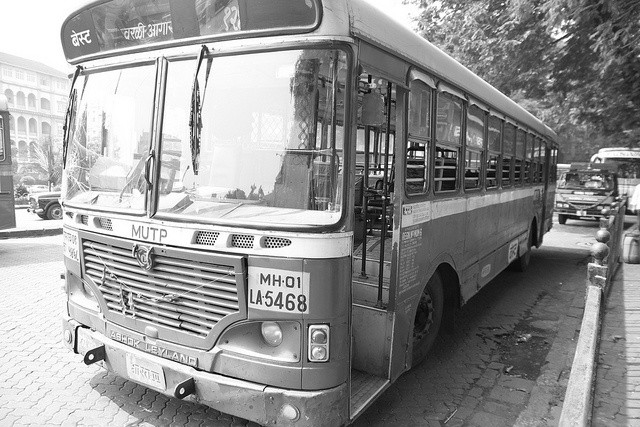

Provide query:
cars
left=555, top=166, right=618, bottom=225
left=28, top=191, right=60, bottom=218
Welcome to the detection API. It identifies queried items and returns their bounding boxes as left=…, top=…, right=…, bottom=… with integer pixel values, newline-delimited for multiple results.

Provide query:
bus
left=57, top=0, right=558, bottom=427
left=591, top=147, right=640, bottom=215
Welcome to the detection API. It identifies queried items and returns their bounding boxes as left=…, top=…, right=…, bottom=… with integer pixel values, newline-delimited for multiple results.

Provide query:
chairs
left=364, top=145, right=444, bottom=232
left=488, top=155, right=543, bottom=184
left=566, top=174, right=580, bottom=186
